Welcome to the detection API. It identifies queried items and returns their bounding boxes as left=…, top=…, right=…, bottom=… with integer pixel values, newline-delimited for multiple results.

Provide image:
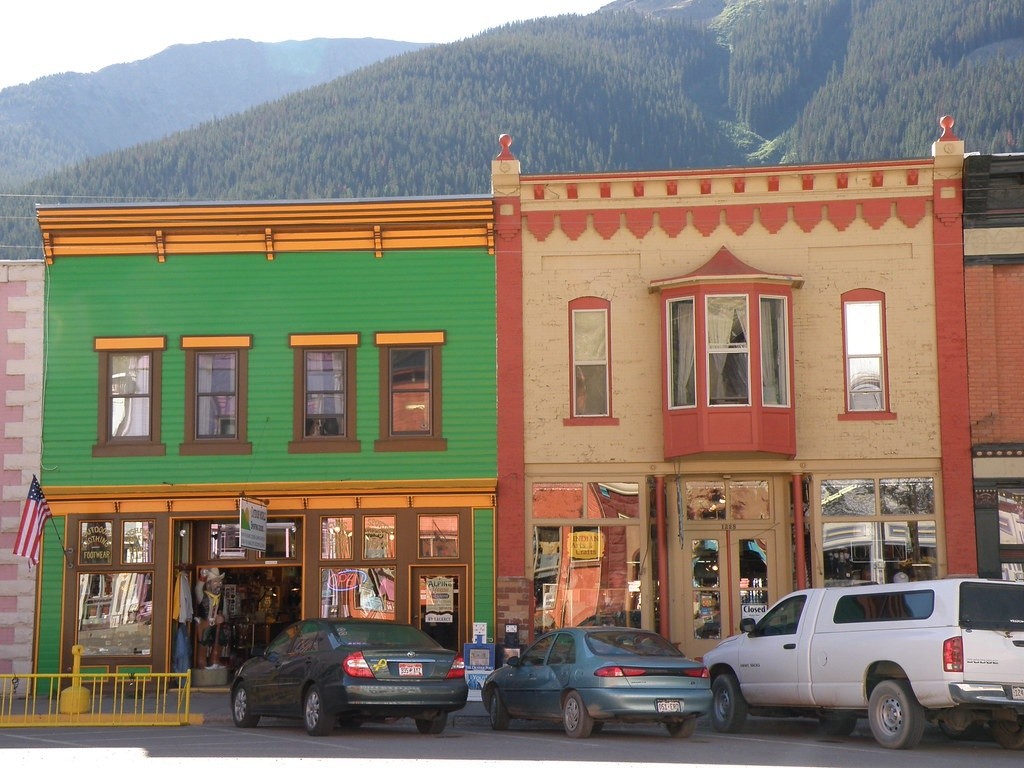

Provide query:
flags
left=13, top=473, right=54, bottom=570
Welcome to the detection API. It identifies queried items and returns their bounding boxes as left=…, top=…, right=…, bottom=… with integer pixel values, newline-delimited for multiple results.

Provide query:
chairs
left=214, top=392, right=237, bottom=436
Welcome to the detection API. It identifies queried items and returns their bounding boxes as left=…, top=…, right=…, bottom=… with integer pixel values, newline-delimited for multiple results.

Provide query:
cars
left=479, top=625, right=716, bottom=739
left=229, top=617, right=469, bottom=736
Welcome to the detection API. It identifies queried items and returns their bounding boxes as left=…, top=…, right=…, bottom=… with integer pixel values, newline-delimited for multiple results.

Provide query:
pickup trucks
left=702, top=577, right=1024, bottom=750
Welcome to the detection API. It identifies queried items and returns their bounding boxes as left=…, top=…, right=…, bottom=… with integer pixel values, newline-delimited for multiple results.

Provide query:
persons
left=194, top=568, right=227, bottom=670
